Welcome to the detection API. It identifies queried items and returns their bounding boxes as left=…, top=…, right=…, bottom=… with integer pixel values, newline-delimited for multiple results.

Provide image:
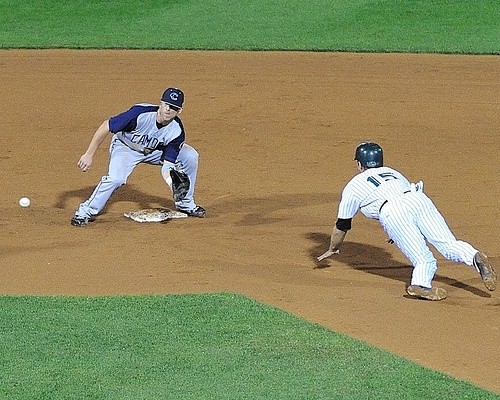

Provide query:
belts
left=377, top=189, right=418, bottom=220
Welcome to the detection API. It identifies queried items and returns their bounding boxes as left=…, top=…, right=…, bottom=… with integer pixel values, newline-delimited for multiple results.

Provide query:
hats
left=161, top=88, right=184, bottom=109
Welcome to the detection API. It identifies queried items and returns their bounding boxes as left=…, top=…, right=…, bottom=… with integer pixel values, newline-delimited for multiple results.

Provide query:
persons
left=72, top=87, right=207, bottom=226
left=317, top=142, right=498, bottom=301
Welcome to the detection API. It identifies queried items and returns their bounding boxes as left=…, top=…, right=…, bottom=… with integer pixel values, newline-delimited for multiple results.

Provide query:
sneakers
left=474, top=252, right=497, bottom=291
left=179, top=205, right=205, bottom=218
left=407, top=285, right=447, bottom=300
left=72, top=215, right=96, bottom=226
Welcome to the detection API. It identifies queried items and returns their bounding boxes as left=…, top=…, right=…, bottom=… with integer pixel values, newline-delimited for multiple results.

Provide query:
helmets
left=354, top=143, right=383, bottom=168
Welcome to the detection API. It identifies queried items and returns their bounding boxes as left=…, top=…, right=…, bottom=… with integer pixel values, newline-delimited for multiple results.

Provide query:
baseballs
left=19, top=197, right=31, bottom=207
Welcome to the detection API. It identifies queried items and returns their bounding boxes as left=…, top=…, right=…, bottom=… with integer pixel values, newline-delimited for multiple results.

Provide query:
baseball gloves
left=169, top=169, right=190, bottom=202
